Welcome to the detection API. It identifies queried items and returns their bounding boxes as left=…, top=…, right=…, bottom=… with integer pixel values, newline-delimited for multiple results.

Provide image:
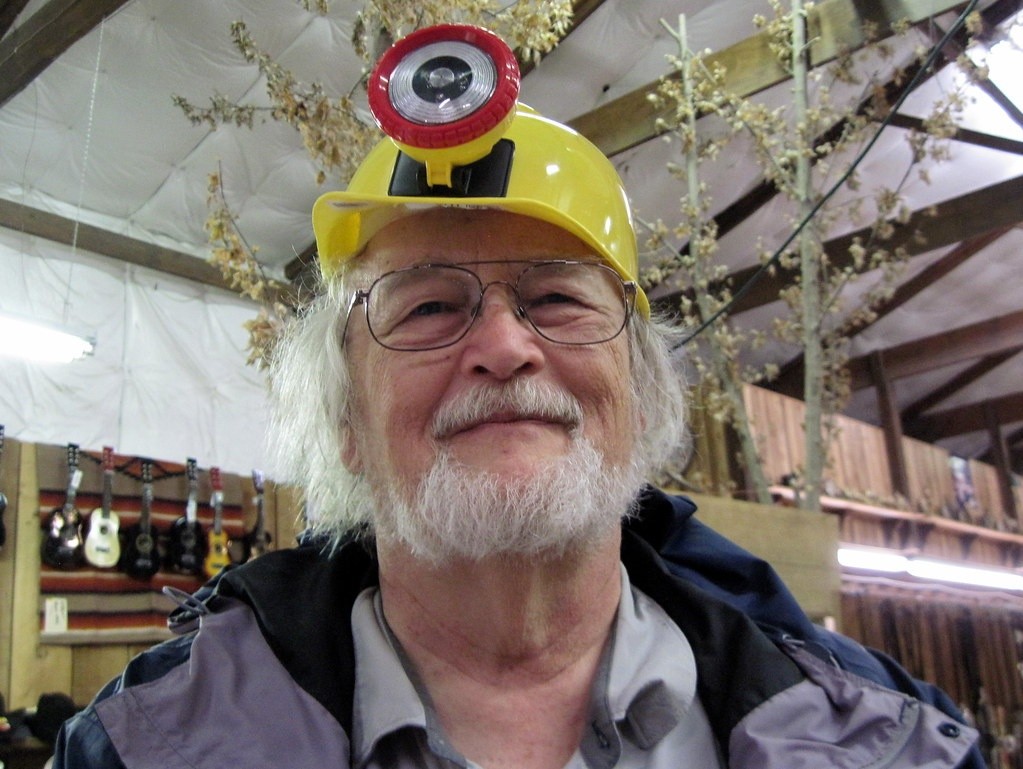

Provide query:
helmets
left=313, top=24, right=650, bottom=326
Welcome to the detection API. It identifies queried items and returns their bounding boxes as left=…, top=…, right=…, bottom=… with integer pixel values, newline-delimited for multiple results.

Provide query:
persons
left=41, top=102, right=986, bottom=768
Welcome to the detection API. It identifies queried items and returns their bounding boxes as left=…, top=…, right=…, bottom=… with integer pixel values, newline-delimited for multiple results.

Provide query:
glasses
left=341, top=261, right=636, bottom=352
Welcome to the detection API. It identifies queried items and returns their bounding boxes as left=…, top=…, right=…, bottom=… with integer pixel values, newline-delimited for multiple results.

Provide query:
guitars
left=121, top=461, right=161, bottom=579
left=0, top=425, right=9, bottom=547
left=80, top=446, right=123, bottom=568
left=165, top=458, right=205, bottom=574
left=241, top=471, right=273, bottom=565
left=203, top=467, right=232, bottom=578
left=43, top=442, right=85, bottom=568
